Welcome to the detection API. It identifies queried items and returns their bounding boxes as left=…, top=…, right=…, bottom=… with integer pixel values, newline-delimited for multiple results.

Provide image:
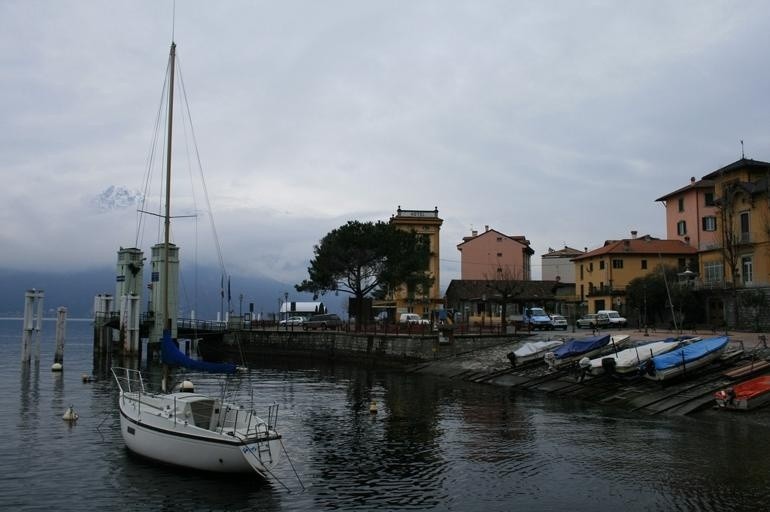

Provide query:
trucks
left=508, top=307, right=551, bottom=331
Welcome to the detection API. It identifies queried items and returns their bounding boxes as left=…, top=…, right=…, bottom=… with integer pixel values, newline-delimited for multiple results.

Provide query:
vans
left=597, top=310, right=627, bottom=329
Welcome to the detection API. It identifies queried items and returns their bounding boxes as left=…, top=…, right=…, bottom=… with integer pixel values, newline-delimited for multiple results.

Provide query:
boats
left=506, top=332, right=770, bottom=413
left=110, top=365, right=283, bottom=480
left=61, top=404, right=79, bottom=421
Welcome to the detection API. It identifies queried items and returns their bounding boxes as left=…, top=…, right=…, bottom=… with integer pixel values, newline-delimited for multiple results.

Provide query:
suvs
left=575, top=314, right=597, bottom=328
left=301, top=313, right=343, bottom=331
left=279, top=316, right=307, bottom=328
left=548, top=313, right=568, bottom=330
left=399, top=313, right=430, bottom=326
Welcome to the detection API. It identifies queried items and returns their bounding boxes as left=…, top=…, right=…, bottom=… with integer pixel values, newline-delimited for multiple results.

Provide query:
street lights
left=284, top=292, right=288, bottom=331
